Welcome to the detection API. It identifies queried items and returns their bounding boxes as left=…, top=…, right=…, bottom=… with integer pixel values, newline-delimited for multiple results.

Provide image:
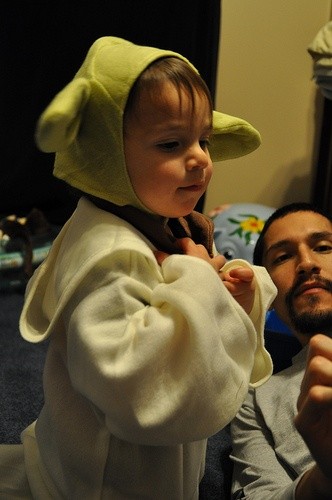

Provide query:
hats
left=36, top=36, right=262, bottom=217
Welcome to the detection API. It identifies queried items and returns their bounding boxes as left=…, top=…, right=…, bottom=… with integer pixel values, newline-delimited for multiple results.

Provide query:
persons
left=20, top=35, right=278, bottom=500
left=225, top=202, right=332, bottom=500
